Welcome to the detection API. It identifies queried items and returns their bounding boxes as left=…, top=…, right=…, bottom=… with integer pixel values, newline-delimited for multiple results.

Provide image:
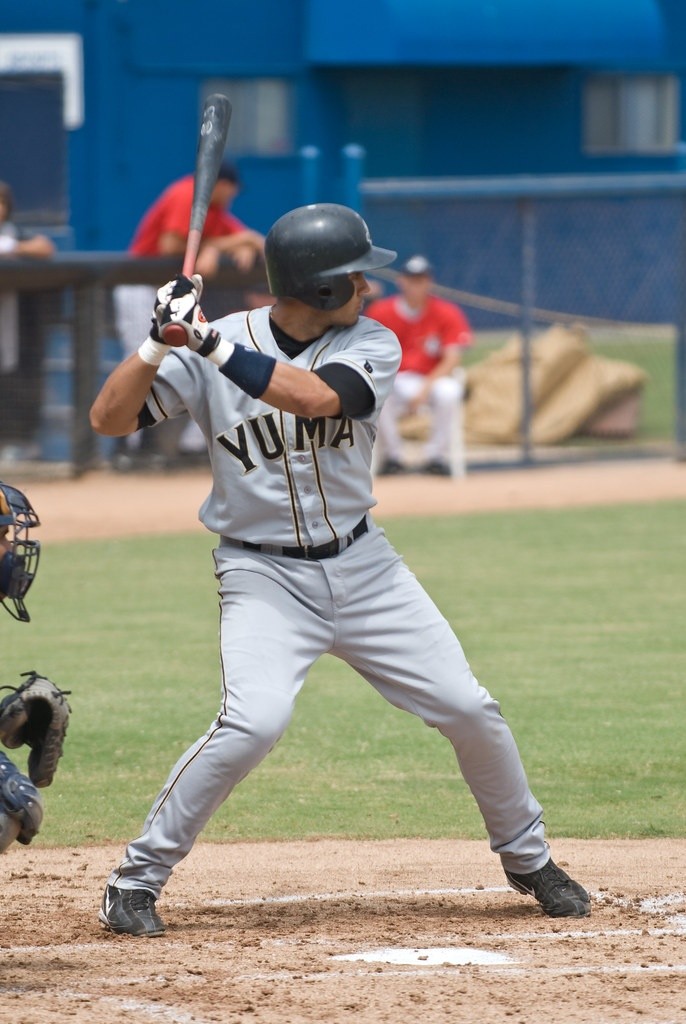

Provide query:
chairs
left=370, top=368, right=465, bottom=480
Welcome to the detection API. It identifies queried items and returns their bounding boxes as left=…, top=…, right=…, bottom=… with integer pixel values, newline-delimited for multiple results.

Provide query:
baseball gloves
left=0, top=670, right=73, bottom=789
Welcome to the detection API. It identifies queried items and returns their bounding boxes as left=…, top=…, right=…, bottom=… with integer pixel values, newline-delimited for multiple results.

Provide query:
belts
left=243, top=514, right=368, bottom=560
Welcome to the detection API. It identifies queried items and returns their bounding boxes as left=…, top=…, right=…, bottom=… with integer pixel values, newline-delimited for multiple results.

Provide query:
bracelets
left=218, top=342, right=277, bottom=399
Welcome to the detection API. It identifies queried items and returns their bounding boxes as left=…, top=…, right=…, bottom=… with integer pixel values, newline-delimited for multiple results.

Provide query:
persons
left=89, top=204, right=594, bottom=937
left=364, top=255, right=473, bottom=479
left=0, top=183, right=55, bottom=462
left=112, top=162, right=265, bottom=455
left=0, top=479, right=72, bottom=855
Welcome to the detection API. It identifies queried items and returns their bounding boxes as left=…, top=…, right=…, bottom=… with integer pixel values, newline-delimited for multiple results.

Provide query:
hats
left=400, top=254, right=432, bottom=276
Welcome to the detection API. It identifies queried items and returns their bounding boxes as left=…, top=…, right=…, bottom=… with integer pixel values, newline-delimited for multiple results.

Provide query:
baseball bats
left=162, top=92, right=235, bottom=348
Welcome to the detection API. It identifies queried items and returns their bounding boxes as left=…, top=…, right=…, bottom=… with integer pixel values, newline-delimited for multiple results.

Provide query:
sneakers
left=503, top=856, right=592, bottom=918
left=98, top=883, right=165, bottom=937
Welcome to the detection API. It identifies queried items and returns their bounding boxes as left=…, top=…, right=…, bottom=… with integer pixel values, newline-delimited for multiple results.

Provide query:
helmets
left=0, top=480, right=41, bottom=622
left=264, top=201, right=398, bottom=310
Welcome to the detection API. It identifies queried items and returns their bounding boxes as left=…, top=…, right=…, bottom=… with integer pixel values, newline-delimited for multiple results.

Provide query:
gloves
left=155, top=274, right=221, bottom=358
left=148, top=274, right=206, bottom=355
